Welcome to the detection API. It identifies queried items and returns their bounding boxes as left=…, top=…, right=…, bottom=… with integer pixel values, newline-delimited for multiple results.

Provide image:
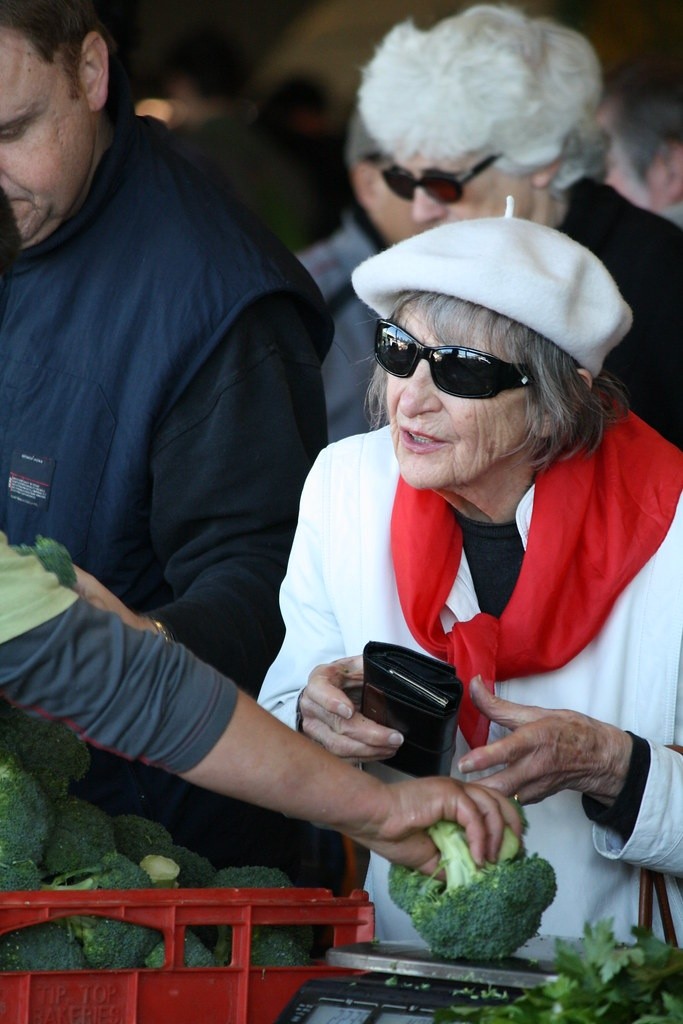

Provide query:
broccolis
left=0, top=535, right=323, bottom=970
left=388, top=795, right=558, bottom=961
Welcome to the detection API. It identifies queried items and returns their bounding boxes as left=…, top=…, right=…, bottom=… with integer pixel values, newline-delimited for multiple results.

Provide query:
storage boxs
left=0, top=886, right=375, bottom=1024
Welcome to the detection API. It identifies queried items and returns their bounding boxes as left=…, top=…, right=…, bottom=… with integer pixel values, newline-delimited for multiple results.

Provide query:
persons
left=0, top=192, right=527, bottom=882
left=253, top=195, right=683, bottom=939
left=0, top=0, right=334, bottom=872
left=296, top=0, right=683, bottom=449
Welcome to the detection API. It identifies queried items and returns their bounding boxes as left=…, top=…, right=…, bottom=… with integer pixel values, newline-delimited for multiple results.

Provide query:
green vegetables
left=429, top=909, right=683, bottom=1024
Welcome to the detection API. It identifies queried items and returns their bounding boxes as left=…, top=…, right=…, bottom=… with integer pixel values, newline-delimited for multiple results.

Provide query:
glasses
left=381, top=152, right=502, bottom=203
left=372, top=318, right=537, bottom=399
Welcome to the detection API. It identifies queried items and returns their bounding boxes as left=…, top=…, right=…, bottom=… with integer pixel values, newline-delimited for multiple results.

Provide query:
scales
left=273, top=934, right=636, bottom=1024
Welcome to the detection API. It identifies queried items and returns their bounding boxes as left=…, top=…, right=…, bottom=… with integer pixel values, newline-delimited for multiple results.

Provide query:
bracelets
left=154, top=620, right=172, bottom=641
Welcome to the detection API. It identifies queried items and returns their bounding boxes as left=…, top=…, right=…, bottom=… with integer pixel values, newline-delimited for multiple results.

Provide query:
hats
left=350, top=216, right=633, bottom=377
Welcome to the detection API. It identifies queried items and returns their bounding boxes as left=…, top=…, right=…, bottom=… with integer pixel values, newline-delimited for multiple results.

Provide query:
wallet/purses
left=360, top=640, right=464, bottom=780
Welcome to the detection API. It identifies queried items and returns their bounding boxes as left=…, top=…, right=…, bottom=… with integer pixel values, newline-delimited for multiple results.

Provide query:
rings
left=513, top=794, right=519, bottom=801
